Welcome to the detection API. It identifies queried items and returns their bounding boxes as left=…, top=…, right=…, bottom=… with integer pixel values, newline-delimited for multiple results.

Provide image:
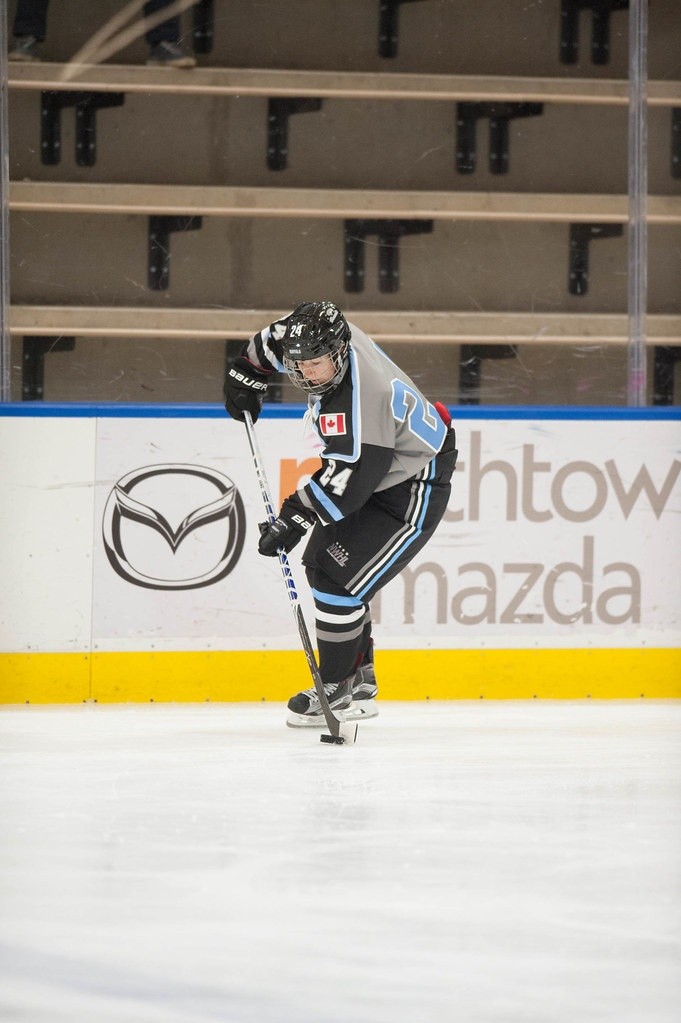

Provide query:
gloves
left=224, top=354, right=274, bottom=424
left=257, top=493, right=317, bottom=557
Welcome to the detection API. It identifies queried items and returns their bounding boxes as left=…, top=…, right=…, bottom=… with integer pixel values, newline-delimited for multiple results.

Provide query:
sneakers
left=286, top=679, right=352, bottom=726
left=344, top=664, right=380, bottom=720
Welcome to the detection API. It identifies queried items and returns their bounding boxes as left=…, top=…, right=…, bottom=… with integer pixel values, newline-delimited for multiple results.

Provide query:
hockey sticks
left=245, top=409, right=359, bottom=741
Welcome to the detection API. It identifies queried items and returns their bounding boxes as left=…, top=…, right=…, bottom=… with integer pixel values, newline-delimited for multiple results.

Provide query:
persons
left=222, top=301, right=458, bottom=728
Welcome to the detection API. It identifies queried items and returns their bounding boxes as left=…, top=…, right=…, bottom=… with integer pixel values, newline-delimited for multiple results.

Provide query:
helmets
left=283, top=302, right=351, bottom=397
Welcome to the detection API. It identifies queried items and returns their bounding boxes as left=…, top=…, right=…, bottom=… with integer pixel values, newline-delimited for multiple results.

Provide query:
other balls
left=321, top=734, right=344, bottom=744
